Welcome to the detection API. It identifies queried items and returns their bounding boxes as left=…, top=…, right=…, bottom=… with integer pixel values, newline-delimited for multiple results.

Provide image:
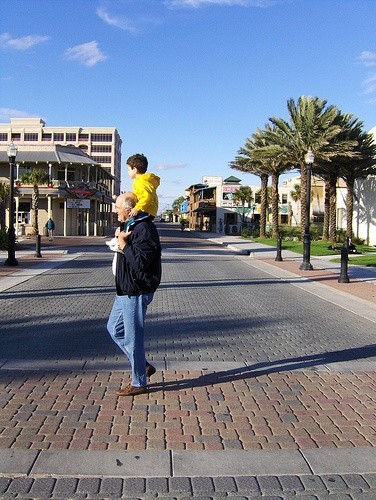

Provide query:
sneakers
left=105, top=237, right=118, bottom=245
left=110, top=244, right=122, bottom=253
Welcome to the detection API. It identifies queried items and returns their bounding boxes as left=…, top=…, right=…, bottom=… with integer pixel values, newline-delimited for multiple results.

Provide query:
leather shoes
left=129, top=365, right=156, bottom=381
left=115, top=382, right=146, bottom=395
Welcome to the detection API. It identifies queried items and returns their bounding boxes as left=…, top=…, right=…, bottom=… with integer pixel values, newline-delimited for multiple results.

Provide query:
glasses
left=115, top=205, right=124, bottom=210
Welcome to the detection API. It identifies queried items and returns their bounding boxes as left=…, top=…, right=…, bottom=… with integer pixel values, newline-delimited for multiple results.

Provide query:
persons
left=105, top=153, right=160, bottom=252
left=180, top=219, right=186, bottom=228
left=106, top=193, right=162, bottom=396
left=45, top=218, right=55, bottom=241
left=218, top=218, right=223, bottom=234
left=205, top=219, right=209, bottom=230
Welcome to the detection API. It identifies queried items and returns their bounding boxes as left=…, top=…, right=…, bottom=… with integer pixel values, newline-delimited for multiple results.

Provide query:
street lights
left=3, top=140, right=19, bottom=268
left=298, top=146, right=315, bottom=271
